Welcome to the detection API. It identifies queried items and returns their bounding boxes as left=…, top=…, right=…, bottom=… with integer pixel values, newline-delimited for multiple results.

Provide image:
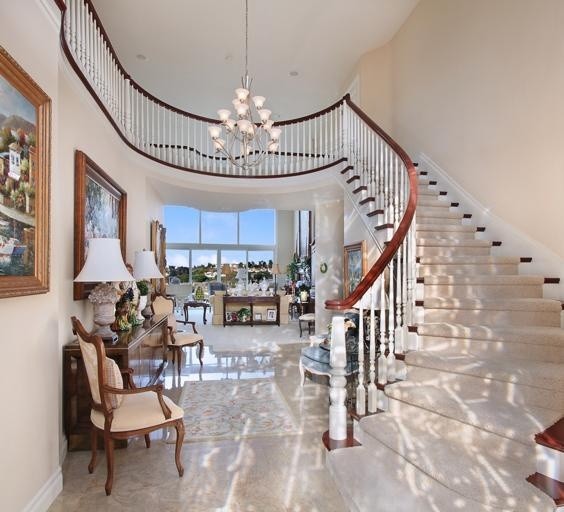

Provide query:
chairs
left=69, top=315, right=185, bottom=496
left=298, top=312, right=369, bottom=388
left=150, top=293, right=204, bottom=375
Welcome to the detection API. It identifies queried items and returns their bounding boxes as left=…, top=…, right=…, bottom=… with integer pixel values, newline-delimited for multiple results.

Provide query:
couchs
left=208, top=291, right=288, bottom=325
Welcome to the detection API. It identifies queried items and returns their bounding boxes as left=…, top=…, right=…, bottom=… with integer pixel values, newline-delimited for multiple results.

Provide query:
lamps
left=132, top=250, right=165, bottom=319
left=351, top=276, right=391, bottom=353
left=73, top=237, right=134, bottom=343
left=271, top=263, right=281, bottom=296
left=221, top=263, right=232, bottom=296
left=207, top=0, right=282, bottom=171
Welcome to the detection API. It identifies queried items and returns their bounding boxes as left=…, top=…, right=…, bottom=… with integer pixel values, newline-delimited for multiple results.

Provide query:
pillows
left=318, top=319, right=358, bottom=352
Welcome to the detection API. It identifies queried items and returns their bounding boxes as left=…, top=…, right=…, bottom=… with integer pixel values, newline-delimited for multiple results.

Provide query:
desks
left=289, top=302, right=307, bottom=320
left=182, top=300, right=207, bottom=325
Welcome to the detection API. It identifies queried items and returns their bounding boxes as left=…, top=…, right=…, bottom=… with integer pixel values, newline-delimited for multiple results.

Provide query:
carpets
left=166, top=377, right=305, bottom=444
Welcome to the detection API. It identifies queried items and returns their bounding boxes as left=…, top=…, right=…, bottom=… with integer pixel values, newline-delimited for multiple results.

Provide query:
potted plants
left=132, top=282, right=148, bottom=321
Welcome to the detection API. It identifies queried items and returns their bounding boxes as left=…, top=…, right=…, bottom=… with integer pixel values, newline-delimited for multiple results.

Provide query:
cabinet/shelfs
left=60, top=314, right=168, bottom=452
left=221, top=293, right=280, bottom=327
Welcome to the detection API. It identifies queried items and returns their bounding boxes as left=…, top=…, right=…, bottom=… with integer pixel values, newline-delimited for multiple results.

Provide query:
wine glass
left=227, top=276, right=274, bottom=297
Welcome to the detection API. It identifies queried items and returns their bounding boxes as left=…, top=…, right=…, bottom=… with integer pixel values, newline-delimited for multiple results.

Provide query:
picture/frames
left=72, top=149, right=127, bottom=301
left=150, top=220, right=166, bottom=296
left=0, top=44, right=53, bottom=300
left=342, top=239, right=367, bottom=301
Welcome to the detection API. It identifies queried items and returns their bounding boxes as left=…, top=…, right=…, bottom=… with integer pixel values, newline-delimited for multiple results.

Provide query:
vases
left=114, top=286, right=134, bottom=332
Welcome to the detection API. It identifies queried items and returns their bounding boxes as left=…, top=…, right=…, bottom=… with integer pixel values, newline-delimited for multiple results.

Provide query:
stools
left=298, top=313, right=315, bottom=338
